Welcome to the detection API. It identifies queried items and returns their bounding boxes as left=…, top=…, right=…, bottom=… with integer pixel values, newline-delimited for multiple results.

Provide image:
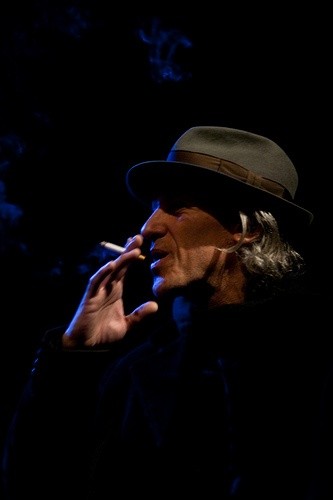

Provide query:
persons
left=0, top=126, right=333, bottom=500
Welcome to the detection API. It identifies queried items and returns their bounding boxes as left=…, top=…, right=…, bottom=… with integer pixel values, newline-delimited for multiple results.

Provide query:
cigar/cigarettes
left=99, top=241, right=147, bottom=261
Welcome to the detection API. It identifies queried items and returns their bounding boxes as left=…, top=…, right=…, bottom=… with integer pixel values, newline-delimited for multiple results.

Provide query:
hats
left=124, top=126, right=315, bottom=227
